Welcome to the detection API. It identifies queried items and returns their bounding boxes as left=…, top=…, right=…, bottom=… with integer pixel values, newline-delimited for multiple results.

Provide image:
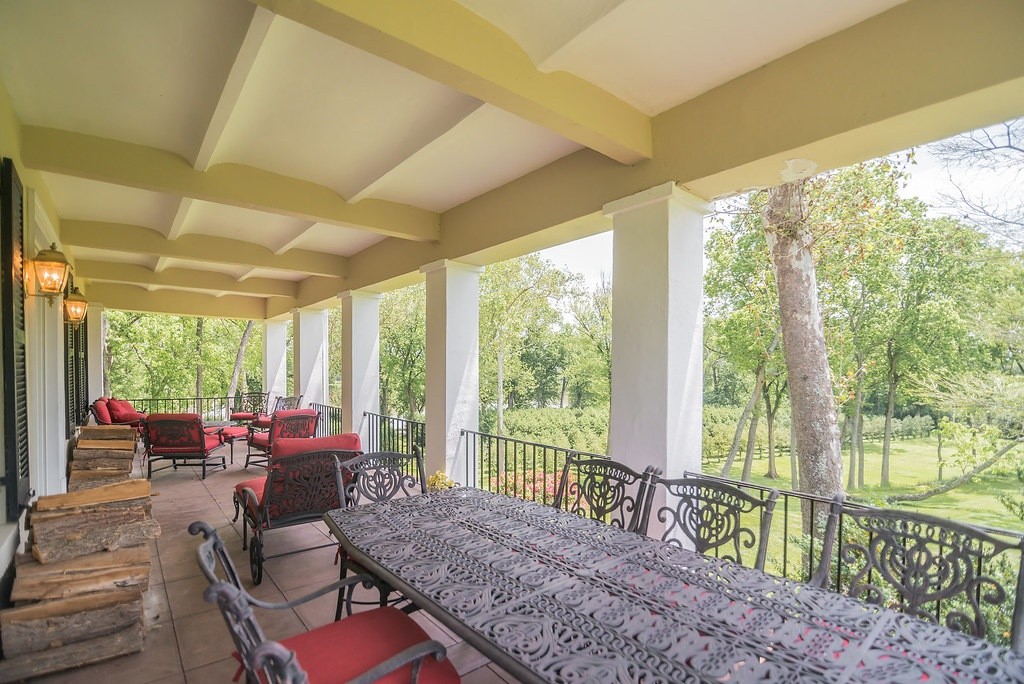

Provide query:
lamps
left=63, top=286, right=91, bottom=331
left=24, top=241, right=71, bottom=308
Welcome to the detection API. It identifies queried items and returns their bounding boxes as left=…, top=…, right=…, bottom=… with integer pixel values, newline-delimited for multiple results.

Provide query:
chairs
left=638, top=466, right=779, bottom=573
left=188, top=520, right=461, bottom=684
left=550, top=450, right=654, bottom=532
left=88, top=389, right=366, bottom=587
left=332, top=445, right=428, bottom=622
left=808, top=493, right=1024, bottom=656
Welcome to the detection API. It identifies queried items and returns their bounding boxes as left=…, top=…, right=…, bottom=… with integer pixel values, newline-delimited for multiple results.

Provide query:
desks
left=203, top=419, right=239, bottom=427
left=322, top=486, right=1024, bottom=684
